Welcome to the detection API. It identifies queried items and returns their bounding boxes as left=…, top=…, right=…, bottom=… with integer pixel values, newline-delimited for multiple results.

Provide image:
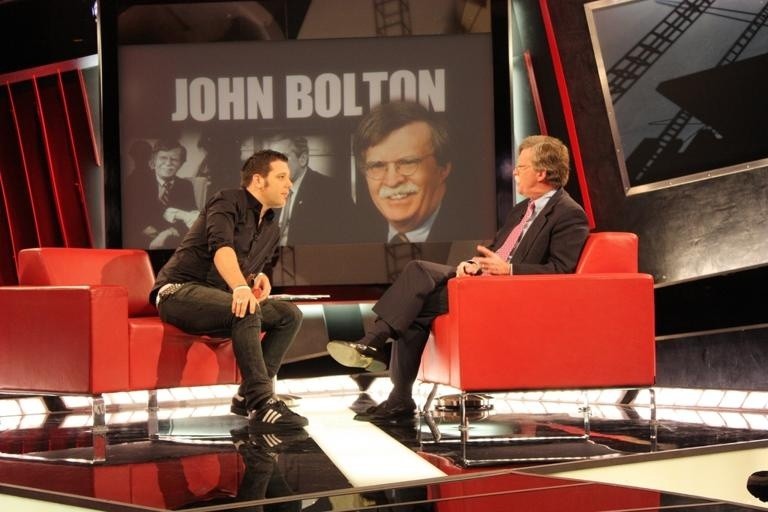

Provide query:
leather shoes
left=354, top=398, right=417, bottom=421
left=327, top=340, right=388, bottom=372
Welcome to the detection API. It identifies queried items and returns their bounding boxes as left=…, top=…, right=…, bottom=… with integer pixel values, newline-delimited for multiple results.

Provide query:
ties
left=159, top=183, right=170, bottom=213
left=481, top=200, right=535, bottom=277
left=390, top=233, right=410, bottom=243
left=280, top=189, right=292, bottom=239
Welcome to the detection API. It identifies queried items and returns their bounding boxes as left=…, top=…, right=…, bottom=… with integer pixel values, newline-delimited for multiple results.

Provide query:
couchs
left=0, top=231, right=264, bottom=467
left=387, top=230, right=657, bottom=469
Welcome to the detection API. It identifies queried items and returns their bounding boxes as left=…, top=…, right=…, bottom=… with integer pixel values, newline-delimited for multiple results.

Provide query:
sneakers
left=248, top=401, right=308, bottom=427
left=230, top=428, right=250, bottom=455
left=232, top=393, right=250, bottom=417
left=248, top=427, right=310, bottom=453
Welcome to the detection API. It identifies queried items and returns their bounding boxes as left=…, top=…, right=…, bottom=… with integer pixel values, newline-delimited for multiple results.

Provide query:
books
left=268, top=294, right=330, bottom=300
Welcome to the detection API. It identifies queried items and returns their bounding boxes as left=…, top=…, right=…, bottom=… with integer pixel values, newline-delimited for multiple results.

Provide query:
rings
left=237, top=299, right=241, bottom=304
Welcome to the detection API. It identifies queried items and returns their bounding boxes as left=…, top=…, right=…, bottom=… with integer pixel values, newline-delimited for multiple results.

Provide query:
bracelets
left=233, top=285, right=251, bottom=291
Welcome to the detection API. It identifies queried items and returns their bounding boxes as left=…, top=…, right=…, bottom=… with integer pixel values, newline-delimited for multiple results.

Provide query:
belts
left=154, top=283, right=172, bottom=312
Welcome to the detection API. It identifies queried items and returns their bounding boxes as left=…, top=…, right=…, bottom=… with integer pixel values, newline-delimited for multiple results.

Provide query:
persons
left=326, top=136, right=589, bottom=423
left=255, top=131, right=342, bottom=284
left=127, top=139, right=200, bottom=248
left=149, top=150, right=308, bottom=429
left=345, top=102, right=489, bottom=282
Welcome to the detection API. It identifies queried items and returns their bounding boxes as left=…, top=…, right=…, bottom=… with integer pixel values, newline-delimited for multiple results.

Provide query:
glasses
left=361, top=151, right=437, bottom=179
left=512, top=162, right=535, bottom=172
